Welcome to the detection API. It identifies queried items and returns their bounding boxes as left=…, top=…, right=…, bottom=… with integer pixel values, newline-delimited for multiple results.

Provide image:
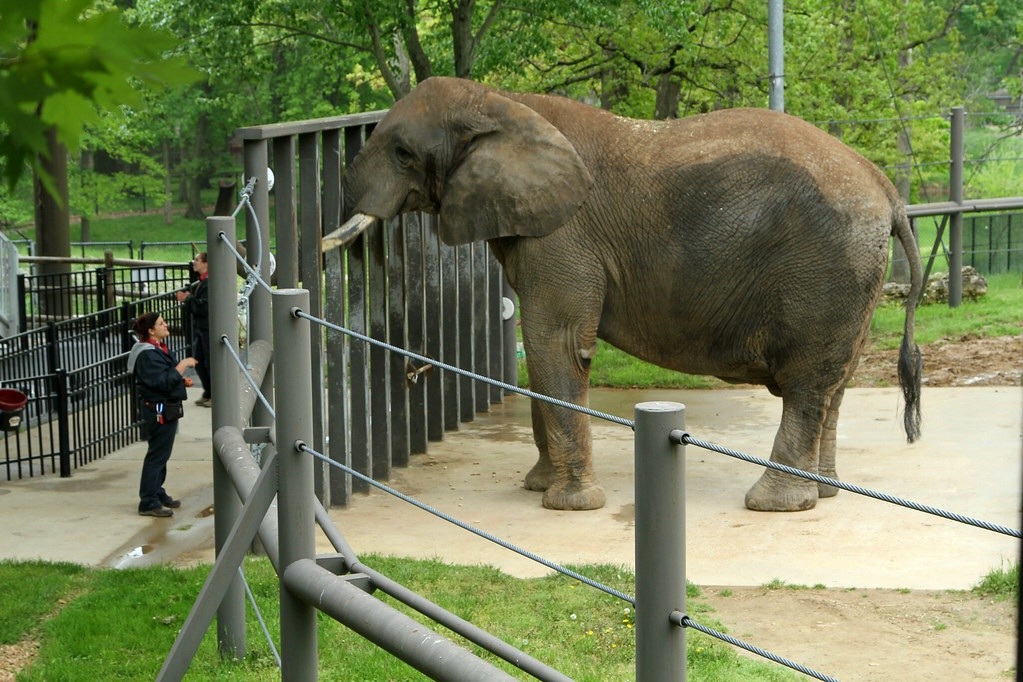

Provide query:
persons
left=127, top=312, right=198, bottom=517
left=177, top=252, right=212, bottom=408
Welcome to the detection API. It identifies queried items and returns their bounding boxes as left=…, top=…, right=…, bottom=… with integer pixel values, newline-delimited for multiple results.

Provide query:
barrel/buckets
left=0, top=388, right=28, bottom=432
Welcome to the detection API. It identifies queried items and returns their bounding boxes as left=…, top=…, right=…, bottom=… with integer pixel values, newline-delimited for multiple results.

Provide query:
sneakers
left=139, top=505, right=172, bottom=517
left=160, top=494, right=180, bottom=508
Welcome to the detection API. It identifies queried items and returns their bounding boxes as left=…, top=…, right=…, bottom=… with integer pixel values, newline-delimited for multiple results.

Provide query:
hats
left=133, top=312, right=159, bottom=334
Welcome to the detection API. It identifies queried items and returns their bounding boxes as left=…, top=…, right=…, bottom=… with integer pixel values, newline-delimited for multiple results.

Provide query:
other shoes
left=204, top=399, right=212, bottom=407
left=195, top=397, right=209, bottom=405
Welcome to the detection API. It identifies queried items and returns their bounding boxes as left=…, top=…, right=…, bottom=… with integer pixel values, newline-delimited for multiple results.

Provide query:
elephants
left=322, top=73, right=928, bottom=517
